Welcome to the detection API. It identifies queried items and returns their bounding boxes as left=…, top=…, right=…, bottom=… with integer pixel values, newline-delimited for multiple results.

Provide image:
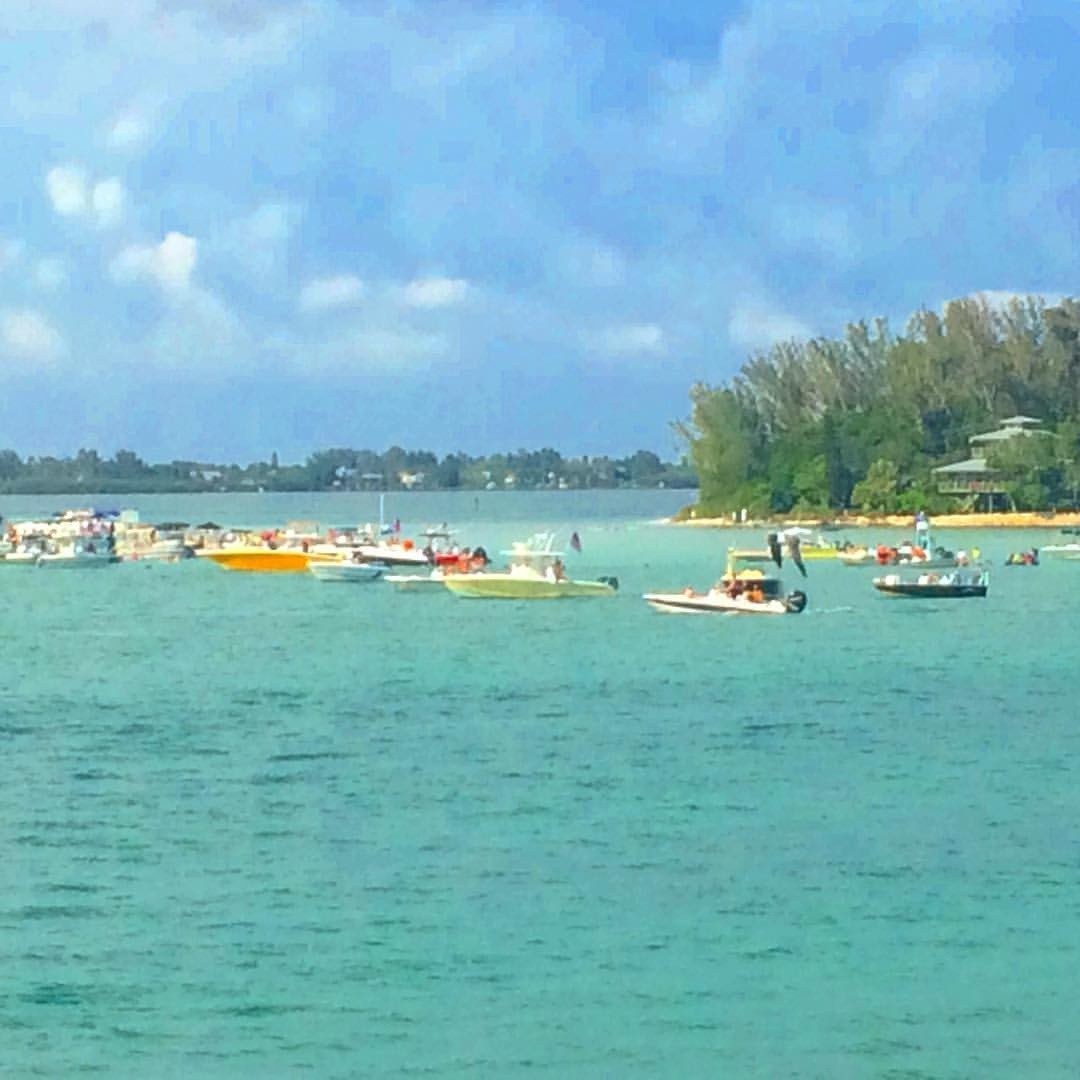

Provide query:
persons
left=954, top=547, right=982, bottom=560
left=421, top=543, right=487, bottom=562
left=685, top=579, right=767, bottom=604
left=919, top=572, right=953, bottom=585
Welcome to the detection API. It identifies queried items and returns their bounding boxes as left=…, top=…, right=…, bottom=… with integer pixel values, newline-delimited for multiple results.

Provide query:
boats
left=306, top=561, right=387, bottom=580
left=10, top=509, right=433, bottom=566
left=441, top=534, right=617, bottom=598
left=1040, top=545, right=1080, bottom=561
left=195, top=548, right=341, bottom=572
left=644, top=570, right=807, bottom=618
left=872, top=568, right=989, bottom=599
left=728, top=543, right=961, bottom=565
